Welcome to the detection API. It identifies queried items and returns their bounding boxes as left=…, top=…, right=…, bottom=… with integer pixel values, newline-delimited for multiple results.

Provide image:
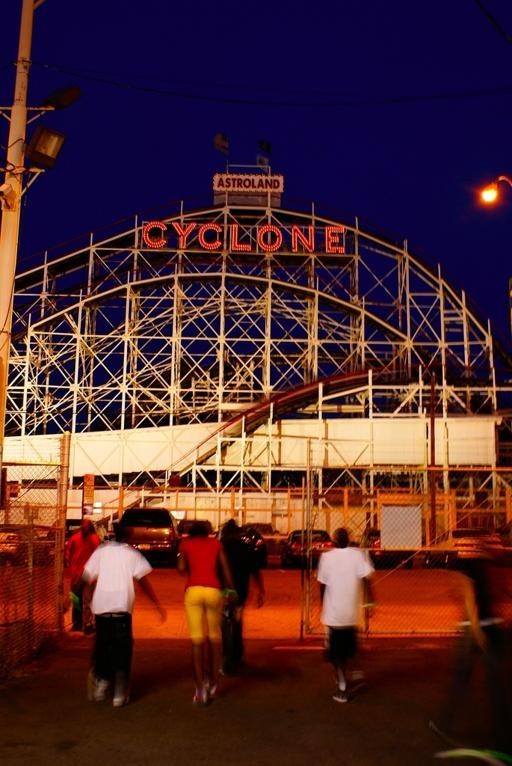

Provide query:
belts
left=99, top=613, right=124, bottom=617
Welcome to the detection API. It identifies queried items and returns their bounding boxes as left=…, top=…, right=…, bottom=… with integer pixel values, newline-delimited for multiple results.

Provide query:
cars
left=358, top=527, right=413, bottom=569
left=424, top=525, right=506, bottom=569
left=178, top=519, right=212, bottom=535
left=243, top=522, right=280, bottom=552
left=279, top=529, right=336, bottom=570
left=0, top=518, right=83, bottom=567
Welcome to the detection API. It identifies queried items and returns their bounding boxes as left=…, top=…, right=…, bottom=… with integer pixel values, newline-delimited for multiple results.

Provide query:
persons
left=316, top=525, right=375, bottom=706
left=82, top=523, right=166, bottom=709
left=174, top=523, right=243, bottom=708
left=63, top=520, right=100, bottom=637
left=216, top=520, right=266, bottom=679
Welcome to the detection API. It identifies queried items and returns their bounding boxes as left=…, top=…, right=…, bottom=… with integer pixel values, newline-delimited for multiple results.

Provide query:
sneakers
left=193, top=680, right=218, bottom=703
left=94, top=679, right=107, bottom=702
left=113, top=694, right=129, bottom=706
left=333, top=694, right=348, bottom=703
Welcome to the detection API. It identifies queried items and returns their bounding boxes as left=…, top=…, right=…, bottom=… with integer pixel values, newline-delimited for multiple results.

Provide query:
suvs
left=112, top=506, right=182, bottom=569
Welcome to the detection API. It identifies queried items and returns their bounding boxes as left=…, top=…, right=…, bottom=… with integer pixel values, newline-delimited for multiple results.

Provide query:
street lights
left=480, top=173, right=512, bottom=208
left=1, top=83, right=81, bottom=461
